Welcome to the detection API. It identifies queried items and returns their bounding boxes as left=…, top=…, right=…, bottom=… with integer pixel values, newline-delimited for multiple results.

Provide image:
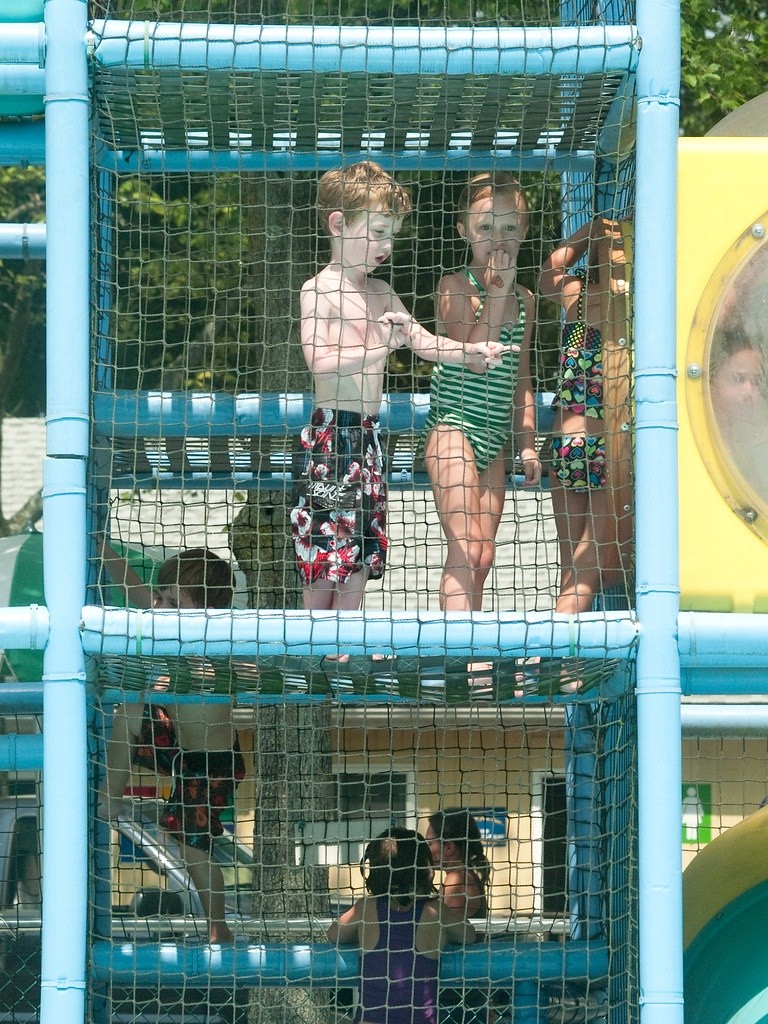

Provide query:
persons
left=425, top=808, right=492, bottom=943
left=534, top=214, right=634, bottom=615
left=552, top=326, right=768, bottom=614
left=292, top=162, right=521, bottom=659
left=419, top=171, right=540, bottom=613
left=327, top=827, right=475, bottom=1024
left=96, top=535, right=246, bottom=943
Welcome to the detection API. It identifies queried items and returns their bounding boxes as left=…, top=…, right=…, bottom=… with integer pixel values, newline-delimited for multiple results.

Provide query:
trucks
left=0, top=795, right=259, bottom=1023
